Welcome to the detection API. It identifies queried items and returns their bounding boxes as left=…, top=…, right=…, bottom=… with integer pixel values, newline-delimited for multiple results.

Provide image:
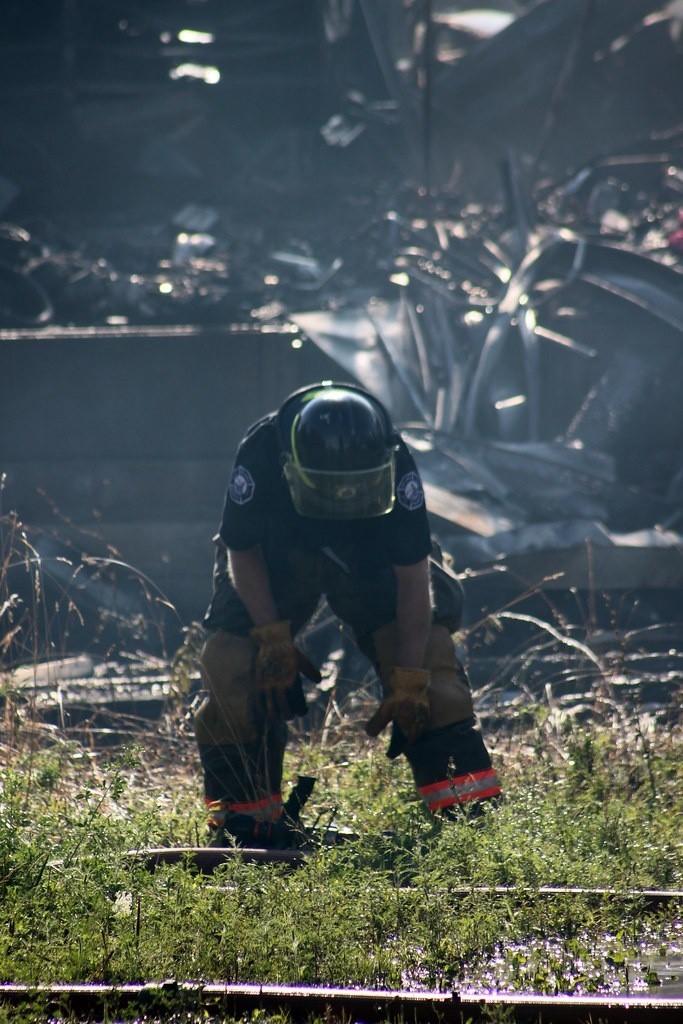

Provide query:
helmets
left=290, top=388, right=392, bottom=514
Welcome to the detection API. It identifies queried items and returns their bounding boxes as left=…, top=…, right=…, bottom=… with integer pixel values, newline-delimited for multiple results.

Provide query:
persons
left=194, top=380, right=499, bottom=871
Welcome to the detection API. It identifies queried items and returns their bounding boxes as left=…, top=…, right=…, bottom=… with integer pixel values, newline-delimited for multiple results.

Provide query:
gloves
left=247, top=620, right=322, bottom=722
left=365, top=665, right=433, bottom=762
